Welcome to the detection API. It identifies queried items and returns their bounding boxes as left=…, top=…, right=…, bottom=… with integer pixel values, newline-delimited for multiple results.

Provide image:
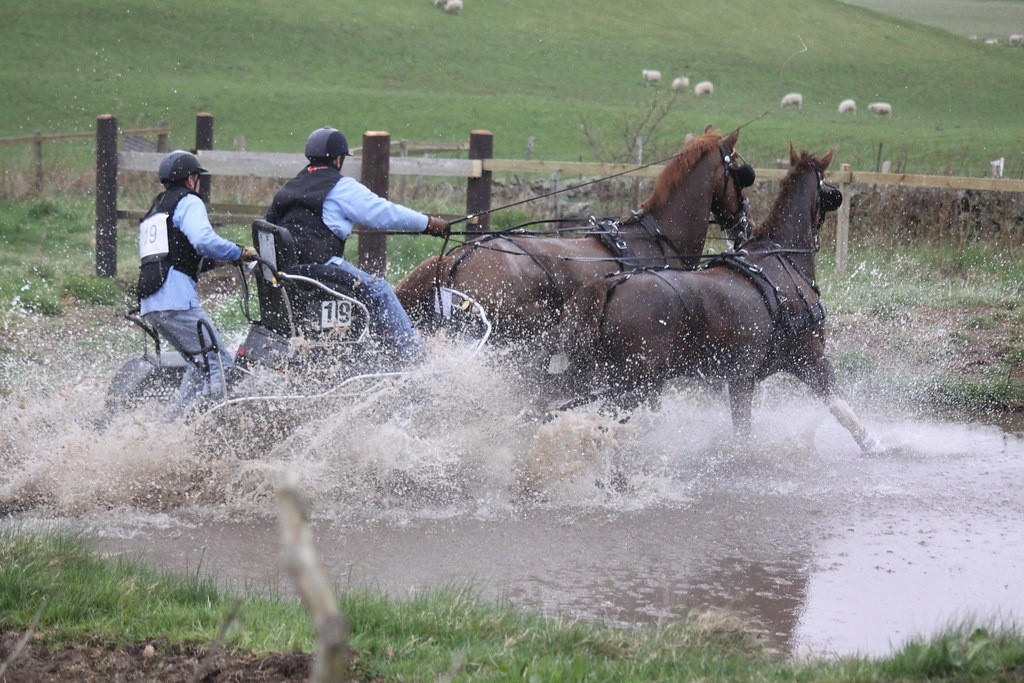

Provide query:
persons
left=264, top=125, right=448, bottom=370
left=136, top=150, right=259, bottom=406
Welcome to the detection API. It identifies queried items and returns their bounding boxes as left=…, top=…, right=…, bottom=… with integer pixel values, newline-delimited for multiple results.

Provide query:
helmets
left=158, top=149, right=208, bottom=192
left=304, top=126, right=353, bottom=171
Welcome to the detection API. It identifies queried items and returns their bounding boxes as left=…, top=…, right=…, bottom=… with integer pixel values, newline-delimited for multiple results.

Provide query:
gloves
left=424, top=214, right=451, bottom=238
left=237, top=244, right=259, bottom=262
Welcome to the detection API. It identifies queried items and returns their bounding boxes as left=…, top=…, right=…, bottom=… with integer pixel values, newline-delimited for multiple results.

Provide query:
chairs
left=251, top=218, right=362, bottom=340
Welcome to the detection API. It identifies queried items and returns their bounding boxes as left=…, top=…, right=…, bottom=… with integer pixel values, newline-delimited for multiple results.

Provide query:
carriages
left=94, top=124, right=888, bottom=461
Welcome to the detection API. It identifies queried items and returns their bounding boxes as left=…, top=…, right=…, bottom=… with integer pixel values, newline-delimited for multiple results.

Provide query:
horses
left=395, top=122, right=890, bottom=458
